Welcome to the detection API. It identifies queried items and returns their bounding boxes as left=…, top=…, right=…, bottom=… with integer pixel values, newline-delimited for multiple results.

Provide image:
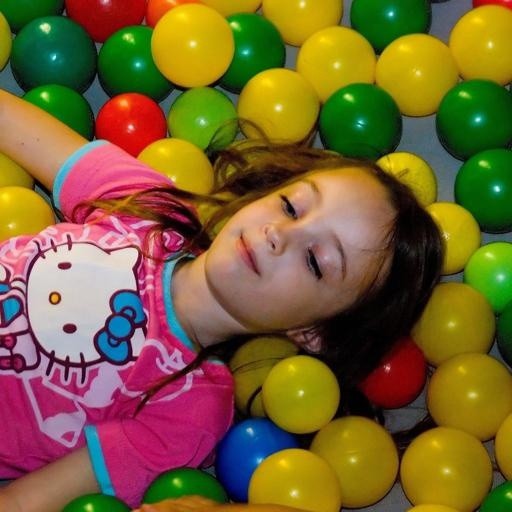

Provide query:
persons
left=0, top=90, right=444, bottom=510
